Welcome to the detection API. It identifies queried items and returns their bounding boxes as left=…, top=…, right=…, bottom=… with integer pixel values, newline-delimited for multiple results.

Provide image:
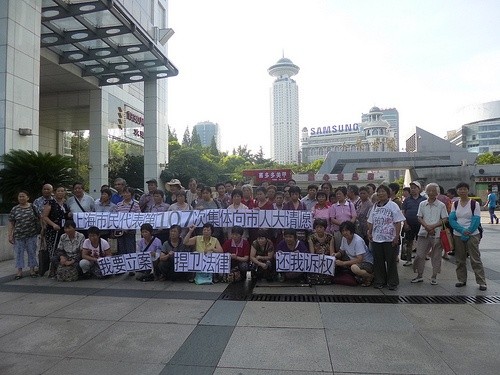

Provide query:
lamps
left=18, top=128, right=34, bottom=137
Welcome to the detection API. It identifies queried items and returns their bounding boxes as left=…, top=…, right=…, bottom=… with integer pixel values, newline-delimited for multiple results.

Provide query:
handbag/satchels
left=336, top=270, right=359, bottom=285
left=31, top=204, right=42, bottom=234
left=109, top=238, right=117, bottom=253
left=440, top=219, right=454, bottom=251
left=194, top=272, right=212, bottom=285
left=37, top=234, right=50, bottom=277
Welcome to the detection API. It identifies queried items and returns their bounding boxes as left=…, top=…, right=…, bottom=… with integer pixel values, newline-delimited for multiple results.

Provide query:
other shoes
left=15, top=273, right=23, bottom=279
left=480, top=284, right=487, bottom=290
left=456, top=281, right=465, bottom=286
left=361, top=279, right=373, bottom=287
left=30, top=273, right=37, bottom=278
left=488, top=222, right=492, bottom=224
left=496, top=218, right=498, bottom=224
left=431, top=279, right=437, bottom=285
left=411, top=277, right=423, bottom=282
left=403, top=261, right=412, bottom=266
left=389, top=285, right=398, bottom=291
left=376, top=283, right=385, bottom=288
left=214, top=274, right=219, bottom=282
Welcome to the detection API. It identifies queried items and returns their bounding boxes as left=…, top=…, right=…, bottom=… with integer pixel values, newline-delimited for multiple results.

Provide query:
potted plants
left=0, top=148, right=77, bottom=225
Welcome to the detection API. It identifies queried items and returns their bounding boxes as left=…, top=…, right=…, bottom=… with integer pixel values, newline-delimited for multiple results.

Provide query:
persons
left=8, top=180, right=457, bottom=289
left=411, top=183, right=448, bottom=285
left=80, top=226, right=111, bottom=279
left=484, top=189, right=498, bottom=224
left=449, top=183, right=487, bottom=290
left=135, top=223, right=162, bottom=281
left=368, top=185, right=406, bottom=291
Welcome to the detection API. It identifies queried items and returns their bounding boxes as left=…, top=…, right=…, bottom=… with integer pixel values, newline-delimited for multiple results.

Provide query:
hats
left=165, top=178, right=184, bottom=193
left=409, top=181, right=422, bottom=190
left=145, top=178, right=157, bottom=184
